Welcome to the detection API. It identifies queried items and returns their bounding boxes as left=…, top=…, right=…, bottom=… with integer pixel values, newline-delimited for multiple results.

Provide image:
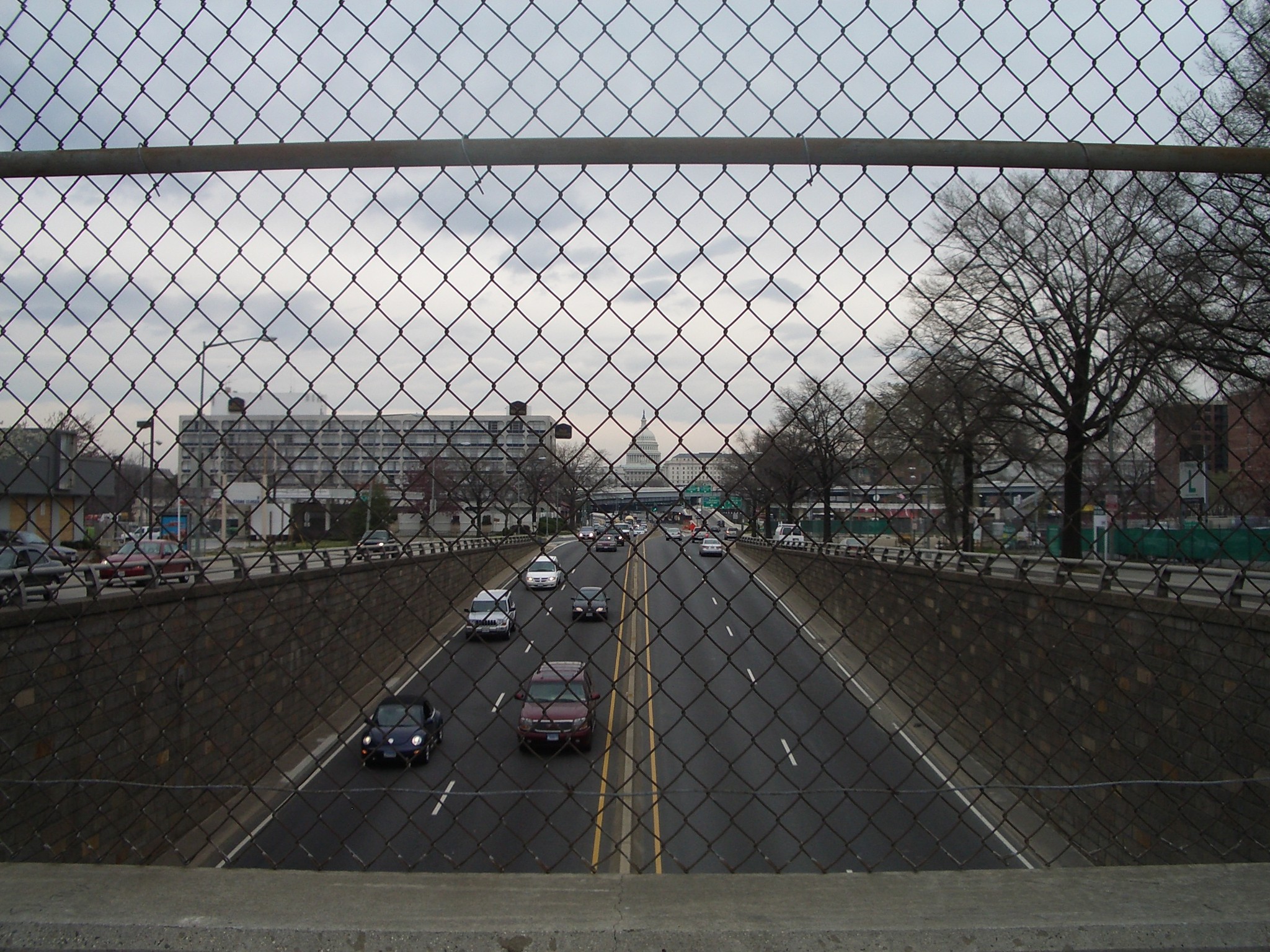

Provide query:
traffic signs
left=684, top=484, right=698, bottom=493
left=699, top=485, right=712, bottom=493
left=724, top=496, right=742, bottom=508
left=703, top=495, right=721, bottom=508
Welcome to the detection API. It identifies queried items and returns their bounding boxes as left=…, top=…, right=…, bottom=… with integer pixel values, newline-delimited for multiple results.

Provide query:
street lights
left=195, top=333, right=279, bottom=558
left=139, top=439, right=164, bottom=538
left=811, top=434, right=853, bottom=538
left=517, top=456, right=547, bottom=534
left=907, top=466, right=932, bottom=559
left=555, top=470, right=582, bottom=537
left=1025, top=315, right=1130, bottom=531
left=365, top=414, right=423, bottom=530
left=136, top=417, right=154, bottom=540
left=429, top=441, right=472, bottom=540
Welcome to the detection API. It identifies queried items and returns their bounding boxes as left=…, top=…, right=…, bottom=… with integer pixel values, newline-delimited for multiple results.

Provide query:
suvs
left=691, top=526, right=709, bottom=543
left=356, top=529, right=400, bottom=561
left=514, top=660, right=601, bottom=753
left=723, top=527, right=739, bottom=540
left=463, top=589, right=517, bottom=641
left=524, top=555, right=562, bottom=590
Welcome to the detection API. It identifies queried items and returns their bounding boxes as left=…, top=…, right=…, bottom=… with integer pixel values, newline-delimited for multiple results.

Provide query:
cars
left=100, top=538, right=191, bottom=589
left=695, top=508, right=725, bottom=533
left=577, top=513, right=648, bottom=552
left=664, top=527, right=683, bottom=542
left=838, top=537, right=869, bottom=559
left=0, top=527, right=80, bottom=607
left=361, top=695, right=445, bottom=770
left=120, top=525, right=161, bottom=544
left=698, top=538, right=723, bottom=557
left=570, top=586, right=611, bottom=621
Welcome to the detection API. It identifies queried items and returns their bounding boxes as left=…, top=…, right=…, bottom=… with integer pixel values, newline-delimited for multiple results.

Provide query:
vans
left=771, top=520, right=805, bottom=547
